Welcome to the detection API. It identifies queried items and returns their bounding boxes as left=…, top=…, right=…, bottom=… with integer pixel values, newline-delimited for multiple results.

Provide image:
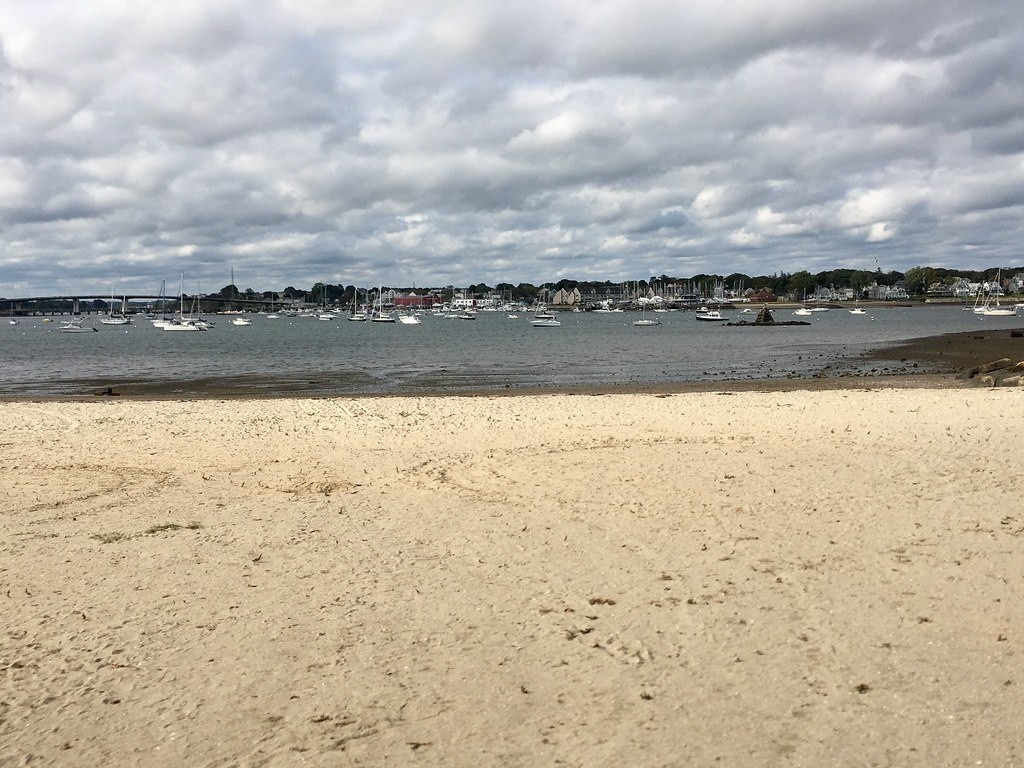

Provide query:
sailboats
left=847, top=299, right=867, bottom=315
left=962, top=267, right=1024, bottom=315
left=104, top=272, right=837, bottom=332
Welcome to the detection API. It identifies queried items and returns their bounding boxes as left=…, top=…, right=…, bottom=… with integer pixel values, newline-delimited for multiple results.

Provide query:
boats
left=9, top=318, right=18, bottom=325
left=58, top=314, right=95, bottom=333
